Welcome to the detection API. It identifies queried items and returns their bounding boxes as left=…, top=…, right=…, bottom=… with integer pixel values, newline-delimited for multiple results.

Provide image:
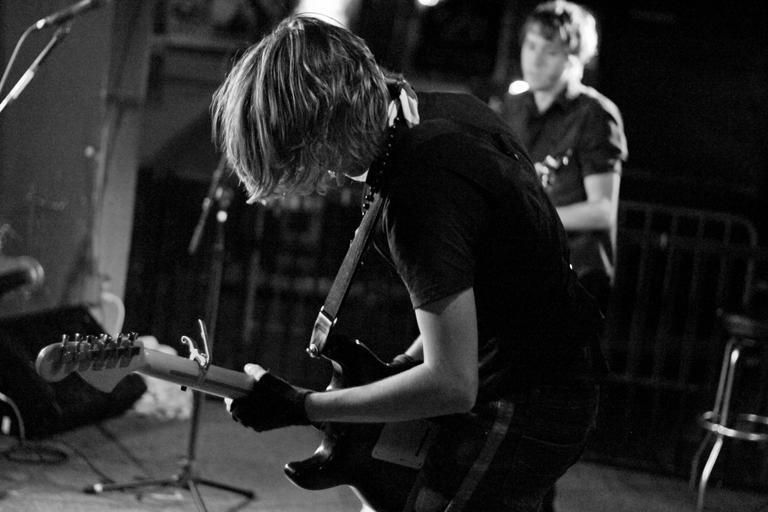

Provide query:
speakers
left=0, top=299, right=147, bottom=439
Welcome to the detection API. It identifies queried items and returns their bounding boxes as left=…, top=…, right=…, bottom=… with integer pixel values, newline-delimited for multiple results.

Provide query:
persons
left=202, top=10, right=600, bottom=512
left=485, top=1, right=627, bottom=307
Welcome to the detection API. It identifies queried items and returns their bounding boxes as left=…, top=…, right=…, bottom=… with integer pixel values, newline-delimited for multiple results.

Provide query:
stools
left=689, top=306, right=768, bottom=511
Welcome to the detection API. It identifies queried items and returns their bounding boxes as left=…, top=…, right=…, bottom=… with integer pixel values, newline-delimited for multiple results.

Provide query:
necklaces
left=359, top=115, right=405, bottom=266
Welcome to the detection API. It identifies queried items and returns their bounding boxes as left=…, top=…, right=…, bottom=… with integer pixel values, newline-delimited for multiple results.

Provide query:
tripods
left=82, top=238, right=256, bottom=512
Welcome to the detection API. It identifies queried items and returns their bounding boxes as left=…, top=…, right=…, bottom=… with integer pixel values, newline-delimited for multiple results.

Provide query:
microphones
left=34, top=1, right=100, bottom=30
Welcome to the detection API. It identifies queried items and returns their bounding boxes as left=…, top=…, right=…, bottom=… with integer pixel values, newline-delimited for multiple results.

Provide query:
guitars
left=36, top=331, right=432, bottom=511
